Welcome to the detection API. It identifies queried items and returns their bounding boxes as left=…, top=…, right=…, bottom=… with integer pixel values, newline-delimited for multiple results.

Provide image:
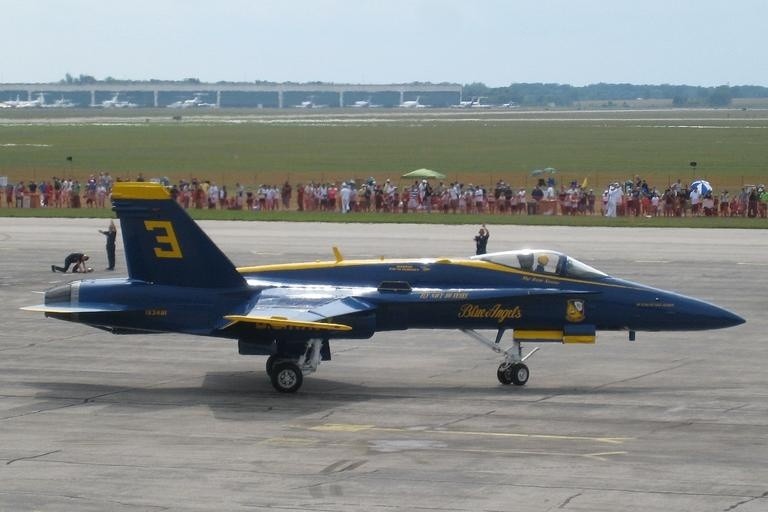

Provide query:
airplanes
left=89, top=93, right=135, bottom=108
left=346, top=98, right=383, bottom=108
left=397, top=96, right=430, bottom=107
left=20, top=182, right=745, bottom=392
left=166, top=93, right=219, bottom=108
left=0, top=93, right=57, bottom=108
left=293, top=97, right=327, bottom=108
left=452, top=97, right=491, bottom=107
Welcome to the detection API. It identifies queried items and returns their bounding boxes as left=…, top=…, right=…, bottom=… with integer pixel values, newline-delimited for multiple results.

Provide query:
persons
left=0, top=170, right=292, bottom=211
left=601, top=174, right=768, bottom=218
left=296, top=174, right=596, bottom=216
left=98, top=220, right=119, bottom=270
left=473, top=220, right=490, bottom=256
left=51, top=252, right=92, bottom=274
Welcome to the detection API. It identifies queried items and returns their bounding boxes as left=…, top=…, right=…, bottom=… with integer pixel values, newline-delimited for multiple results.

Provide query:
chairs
left=518, top=253, right=537, bottom=272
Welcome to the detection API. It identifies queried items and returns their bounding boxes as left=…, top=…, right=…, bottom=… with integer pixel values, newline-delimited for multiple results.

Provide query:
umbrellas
left=400, top=168, right=447, bottom=182
left=530, top=167, right=558, bottom=182
